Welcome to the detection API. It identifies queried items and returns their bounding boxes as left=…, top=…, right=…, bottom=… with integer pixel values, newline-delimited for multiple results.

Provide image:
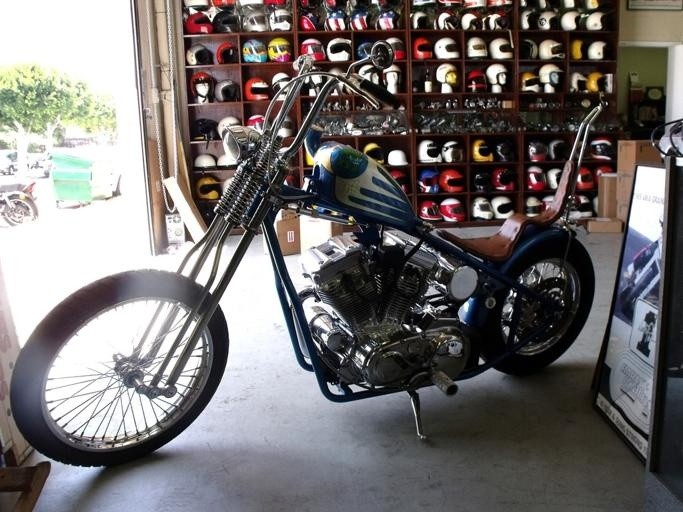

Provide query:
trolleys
left=50, top=154, right=92, bottom=209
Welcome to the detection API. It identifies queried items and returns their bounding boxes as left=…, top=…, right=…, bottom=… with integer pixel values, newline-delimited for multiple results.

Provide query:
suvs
left=106, top=157, right=123, bottom=200
left=44, top=155, right=52, bottom=177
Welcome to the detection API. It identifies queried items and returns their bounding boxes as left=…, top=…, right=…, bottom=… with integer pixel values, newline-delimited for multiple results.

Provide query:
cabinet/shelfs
left=175, top=0, right=302, bottom=213
left=295, top=0, right=414, bottom=222
left=517, top=0, right=619, bottom=224
left=628, top=99, right=665, bottom=140
left=407, top=0, right=523, bottom=228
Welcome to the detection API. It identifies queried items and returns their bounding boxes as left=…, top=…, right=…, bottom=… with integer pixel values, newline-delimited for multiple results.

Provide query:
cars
left=27, top=151, right=52, bottom=169
left=0, top=150, right=28, bottom=175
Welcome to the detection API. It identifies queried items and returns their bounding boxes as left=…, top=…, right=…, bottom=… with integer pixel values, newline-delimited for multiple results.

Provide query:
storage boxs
left=587, top=141, right=662, bottom=232
left=273, top=210, right=344, bottom=254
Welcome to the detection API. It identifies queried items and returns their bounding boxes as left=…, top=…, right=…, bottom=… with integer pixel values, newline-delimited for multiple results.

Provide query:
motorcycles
left=0, top=181, right=38, bottom=227
left=9, top=40, right=608, bottom=468
